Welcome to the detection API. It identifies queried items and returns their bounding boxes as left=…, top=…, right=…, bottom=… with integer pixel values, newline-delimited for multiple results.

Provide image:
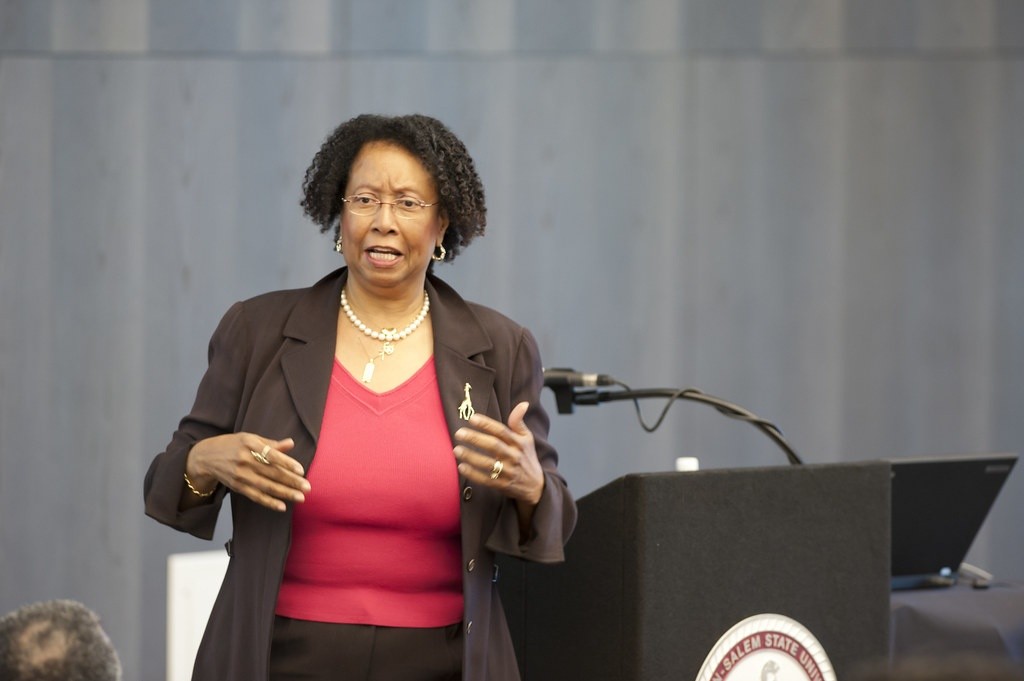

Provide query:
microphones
left=541, top=368, right=614, bottom=387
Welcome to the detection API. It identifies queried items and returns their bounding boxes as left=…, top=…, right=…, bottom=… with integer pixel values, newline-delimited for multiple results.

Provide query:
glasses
left=341, top=196, right=442, bottom=218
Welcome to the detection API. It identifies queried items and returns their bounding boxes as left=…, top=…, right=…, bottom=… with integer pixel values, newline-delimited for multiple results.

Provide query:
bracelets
left=184, top=474, right=216, bottom=498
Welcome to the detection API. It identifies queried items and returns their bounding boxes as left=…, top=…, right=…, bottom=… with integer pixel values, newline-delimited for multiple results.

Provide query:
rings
left=490, top=461, right=503, bottom=480
left=250, top=445, right=272, bottom=464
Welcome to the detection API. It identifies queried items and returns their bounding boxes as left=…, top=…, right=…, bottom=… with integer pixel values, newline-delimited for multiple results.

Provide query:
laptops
left=886, top=454, right=1019, bottom=587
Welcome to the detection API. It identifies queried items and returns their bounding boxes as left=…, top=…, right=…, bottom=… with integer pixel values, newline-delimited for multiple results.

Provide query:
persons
left=142, top=113, right=579, bottom=681
left=0, top=599, right=123, bottom=681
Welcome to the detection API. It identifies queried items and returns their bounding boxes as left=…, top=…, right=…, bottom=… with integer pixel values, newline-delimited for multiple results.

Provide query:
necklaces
left=341, top=289, right=430, bottom=384
